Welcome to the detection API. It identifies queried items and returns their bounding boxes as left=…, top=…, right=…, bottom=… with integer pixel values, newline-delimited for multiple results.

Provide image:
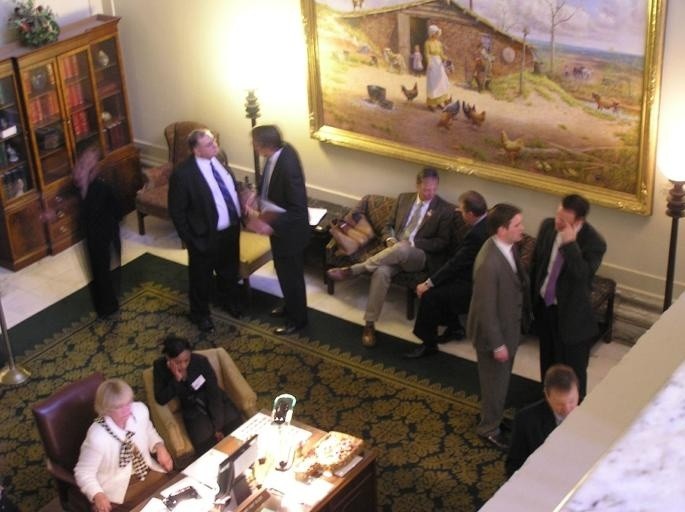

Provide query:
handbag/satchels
left=329, top=211, right=375, bottom=254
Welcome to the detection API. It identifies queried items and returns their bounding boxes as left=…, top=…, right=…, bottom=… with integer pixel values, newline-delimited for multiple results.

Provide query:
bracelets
left=425, top=280, right=431, bottom=289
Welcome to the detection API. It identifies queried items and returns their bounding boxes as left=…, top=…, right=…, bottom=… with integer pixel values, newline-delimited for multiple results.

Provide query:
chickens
left=500, top=130, right=523, bottom=162
left=435, top=95, right=486, bottom=130
left=401, top=81, right=418, bottom=103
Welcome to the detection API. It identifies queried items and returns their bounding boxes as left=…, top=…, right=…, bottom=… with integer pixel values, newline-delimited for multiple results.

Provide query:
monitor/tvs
left=215, top=434, right=260, bottom=512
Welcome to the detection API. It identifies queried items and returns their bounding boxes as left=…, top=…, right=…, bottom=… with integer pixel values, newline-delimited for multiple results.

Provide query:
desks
left=237, top=196, right=351, bottom=311
left=111, top=409, right=376, bottom=512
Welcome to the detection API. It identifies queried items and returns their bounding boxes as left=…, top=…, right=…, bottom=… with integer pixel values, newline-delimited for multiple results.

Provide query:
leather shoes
left=196, top=304, right=466, bottom=359
left=484, top=434, right=511, bottom=450
left=328, top=266, right=353, bottom=281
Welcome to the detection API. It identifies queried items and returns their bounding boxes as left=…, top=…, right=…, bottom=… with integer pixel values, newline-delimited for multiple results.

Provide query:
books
left=254, top=201, right=286, bottom=226
left=1, top=45, right=129, bottom=201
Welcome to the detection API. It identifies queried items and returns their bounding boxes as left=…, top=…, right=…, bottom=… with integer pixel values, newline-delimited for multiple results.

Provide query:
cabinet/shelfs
left=1, top=15, right=141, bottom=271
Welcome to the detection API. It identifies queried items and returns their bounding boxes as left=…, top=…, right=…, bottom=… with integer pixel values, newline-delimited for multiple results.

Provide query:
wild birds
left=369, top=55, right=380, bottom=70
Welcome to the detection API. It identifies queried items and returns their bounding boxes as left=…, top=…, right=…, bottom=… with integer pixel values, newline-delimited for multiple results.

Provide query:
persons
left=525, top=195, right=607, bottom=406
left=327, top=169, right=458, bottom=349
left=169, top=126, right=246, bottom=332
left=55, top=134, right=130, bottom=324
left=424, top=24, right=455, bottom=108
left=405, top=191, right=493, bottom=359
left=411, top=44, right=423, bottom=77
left=248, top=124, right=308, bottom=335
left=465, top=203, right=525, bottom=451
left=73, top=377, right=178, bottom=511
left=504, top=363, right=580, bottom=480
left=153, top=335, right=244, bottom=453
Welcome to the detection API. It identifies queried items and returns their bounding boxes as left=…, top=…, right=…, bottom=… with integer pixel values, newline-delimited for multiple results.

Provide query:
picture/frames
left=297, top=1, right=667, bottom=214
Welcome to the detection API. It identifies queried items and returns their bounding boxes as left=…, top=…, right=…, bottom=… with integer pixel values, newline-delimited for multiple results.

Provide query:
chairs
left=135, top=121, right=230, bottom=249
left=326, top=195, right=537, bottom=337
left=30, top=347, right=259, bottom=511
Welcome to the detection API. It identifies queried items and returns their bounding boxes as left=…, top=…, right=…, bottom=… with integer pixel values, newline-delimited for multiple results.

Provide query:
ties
left=210, top=162, right=239, bottom=225
left=545, top=250, right=564, bottom=307
left=404, top=204, right=423, bottom=242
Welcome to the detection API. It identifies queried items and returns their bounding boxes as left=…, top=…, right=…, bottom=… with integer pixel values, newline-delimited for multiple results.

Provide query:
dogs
left=383, top=48, right=407, bottom=75
left=592, top=91, right=621, bottom=116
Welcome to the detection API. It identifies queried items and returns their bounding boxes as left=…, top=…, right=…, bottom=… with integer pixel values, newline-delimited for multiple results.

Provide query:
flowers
left=6, top=1, right=60, bottom=49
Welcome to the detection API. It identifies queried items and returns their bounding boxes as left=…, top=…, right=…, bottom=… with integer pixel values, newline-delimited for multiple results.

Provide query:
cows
left=472, top=64, right=493, bottom=94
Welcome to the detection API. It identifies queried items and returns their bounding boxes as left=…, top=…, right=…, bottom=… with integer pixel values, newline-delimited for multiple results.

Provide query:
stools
left=571, top=272, right=616, bottom=369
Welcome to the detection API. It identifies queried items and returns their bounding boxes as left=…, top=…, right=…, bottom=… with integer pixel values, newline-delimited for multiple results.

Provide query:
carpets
left=1, top=252, right=540, bottom=512
